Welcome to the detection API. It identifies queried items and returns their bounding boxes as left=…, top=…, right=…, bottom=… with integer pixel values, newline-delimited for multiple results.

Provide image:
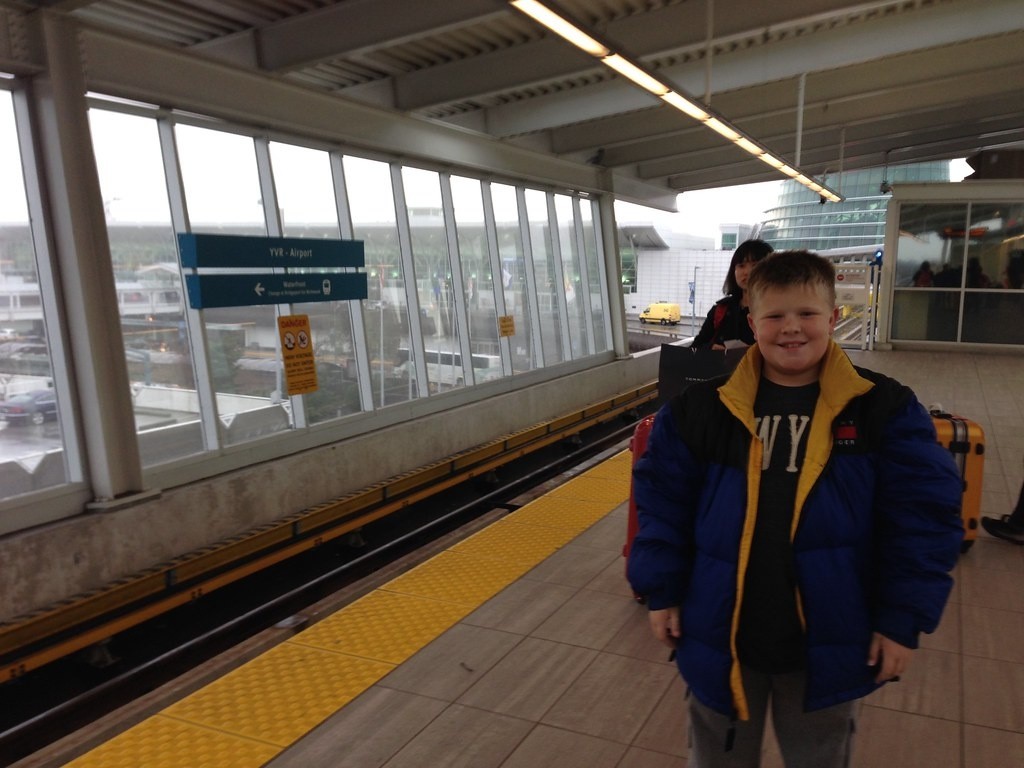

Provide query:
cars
left=0, top=389, right=57, bottom=426
left=315, top=361, right=356, bottom=382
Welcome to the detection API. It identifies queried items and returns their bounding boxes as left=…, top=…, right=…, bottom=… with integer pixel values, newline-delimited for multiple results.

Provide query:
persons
left=623, top=249, right=968, bottom=768
left=909, top=235, right=1023, bottom=345
left=691, top=238, right=787, bottom=355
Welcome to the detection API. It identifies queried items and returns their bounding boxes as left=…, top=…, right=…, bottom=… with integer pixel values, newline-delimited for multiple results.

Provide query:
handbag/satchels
left=658, top=342, right=751, bottom=407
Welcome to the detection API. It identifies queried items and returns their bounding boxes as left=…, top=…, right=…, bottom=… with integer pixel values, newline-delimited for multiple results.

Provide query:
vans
left=639, top=302, right=681, bottom=326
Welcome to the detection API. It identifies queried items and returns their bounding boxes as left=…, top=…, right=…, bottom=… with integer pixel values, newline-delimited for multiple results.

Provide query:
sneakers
left=982, top=514, right=1024, bottom=545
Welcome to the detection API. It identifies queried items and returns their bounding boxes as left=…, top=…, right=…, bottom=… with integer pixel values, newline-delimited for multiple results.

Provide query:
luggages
left=925, top=403, right=984, bottom=554
left=622, top=415, right=667, bottom=602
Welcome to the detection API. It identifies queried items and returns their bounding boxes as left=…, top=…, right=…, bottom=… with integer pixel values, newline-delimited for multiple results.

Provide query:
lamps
left=506, top=0, right=846, bottom=207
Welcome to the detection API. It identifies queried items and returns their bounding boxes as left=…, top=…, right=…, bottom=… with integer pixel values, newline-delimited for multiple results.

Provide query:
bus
left=392, top=348, right=504, bottom=387
